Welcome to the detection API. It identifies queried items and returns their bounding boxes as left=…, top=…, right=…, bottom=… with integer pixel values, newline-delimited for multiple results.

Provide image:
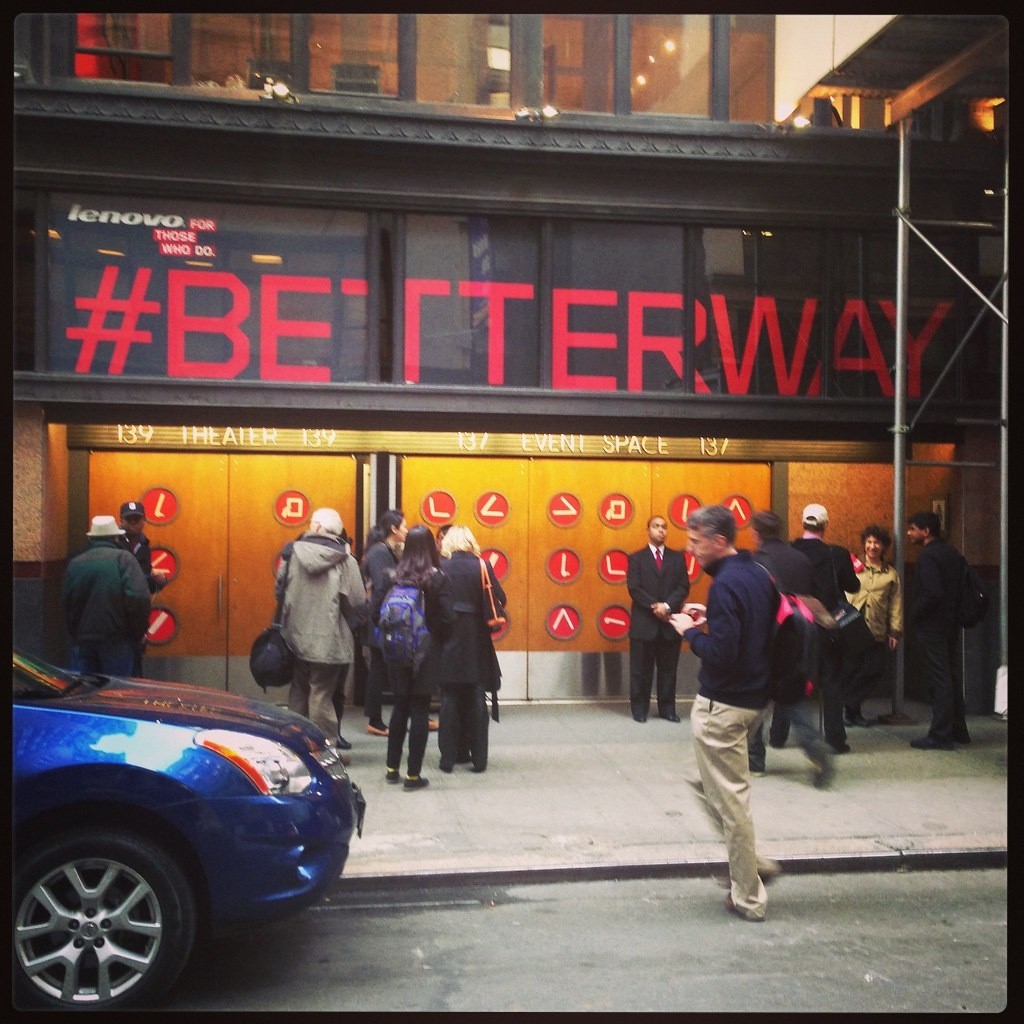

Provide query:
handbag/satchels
left=825, top=601, right=865, bottom=641
left=250, top=625, right=295, bottom=694
left=480, top=557, right=510, bottom=641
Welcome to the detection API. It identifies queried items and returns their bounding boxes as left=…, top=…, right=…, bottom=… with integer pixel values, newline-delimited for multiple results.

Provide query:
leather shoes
left=337, top=735, right=351, bottom=748
left=634, top=716, right=645, bottom=722
left=663, top=714, right=680, bottom=722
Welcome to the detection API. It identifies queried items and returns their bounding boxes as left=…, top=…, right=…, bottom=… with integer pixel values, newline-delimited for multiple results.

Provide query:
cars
left=12, top=646, right=366, bottom=1012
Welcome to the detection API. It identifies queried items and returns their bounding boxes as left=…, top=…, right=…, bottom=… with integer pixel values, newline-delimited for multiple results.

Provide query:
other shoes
left=911, top=736, right=971, bottom=749
left=727, top=893, right=766, bottom=922
left=749, top=769, right=766, bottom=777
left=428, top=720, right=439, bottom=731
left=340, top=754, right=351, bottom=767
left=843, top=717, right=871, bottom=727
left=815, top=753, right=839, bottom=788
left=721, top=871, right=771, bottom=890
left=829, top=745, right=851, bottom=754
left=368, top=723, right=390, bottom=735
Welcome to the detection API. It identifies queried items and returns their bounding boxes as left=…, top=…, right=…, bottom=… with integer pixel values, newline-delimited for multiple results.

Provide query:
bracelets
left=664, top=603, right=671, bottom=611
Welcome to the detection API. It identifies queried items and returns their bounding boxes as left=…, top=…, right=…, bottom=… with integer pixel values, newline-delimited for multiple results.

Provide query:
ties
left=655, top=548, right=662, bottom=573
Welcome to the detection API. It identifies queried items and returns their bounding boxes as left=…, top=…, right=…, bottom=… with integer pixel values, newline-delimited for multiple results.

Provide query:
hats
left=121, top=501, right=144, bottom=517
left=86, top=516, right=126, bottom=535
left=309, top=508, right=343, bottom=537
left=802, top=503, right=829, bottom=526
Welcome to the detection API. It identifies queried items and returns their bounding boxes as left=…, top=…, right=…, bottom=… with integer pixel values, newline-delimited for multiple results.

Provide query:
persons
left=276, top=508, right=508, bottom=790
left=61, top=501, right=159, bottom=682
left=669, top=505, right=783, bottom=920
left=747, top=504, right=903, bottom=789
left=625, top=514, right=690, bottom=721
left=904, top=512, right=971, bottom=750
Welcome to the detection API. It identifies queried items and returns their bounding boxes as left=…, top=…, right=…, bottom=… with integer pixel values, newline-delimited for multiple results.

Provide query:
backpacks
left=957, top=553, right=989, bottom=630
left=378, top=567, right=439, bottom=667
left=753, top=559, right=815, bottom=702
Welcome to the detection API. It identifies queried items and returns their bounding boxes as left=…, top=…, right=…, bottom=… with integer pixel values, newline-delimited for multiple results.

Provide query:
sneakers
left=403, top=775, right=429, bottom=791
left=385, top=767, right=402, bottom=782
